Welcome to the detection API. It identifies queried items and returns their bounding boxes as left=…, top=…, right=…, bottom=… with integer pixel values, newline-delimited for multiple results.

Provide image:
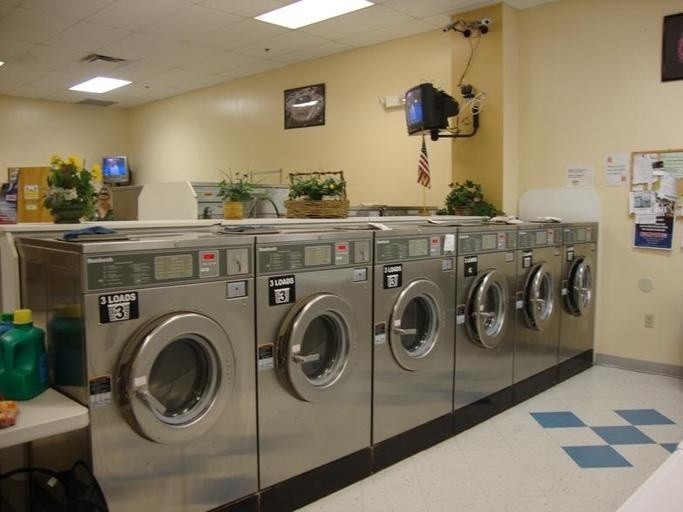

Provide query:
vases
left=51, top=203, right=82, bottom=223
left=224, top=201, right=243, bottom=219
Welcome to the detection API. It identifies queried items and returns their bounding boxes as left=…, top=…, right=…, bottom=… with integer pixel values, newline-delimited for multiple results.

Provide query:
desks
left=0, top=389, right=89, bottom=512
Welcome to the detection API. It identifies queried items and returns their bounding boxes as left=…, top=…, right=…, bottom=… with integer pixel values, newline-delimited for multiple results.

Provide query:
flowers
left=215, top=167, right=257, bottom=203
left=41, top=155, right=104, bottom=217
left=289, top=172, right=344, bottom=199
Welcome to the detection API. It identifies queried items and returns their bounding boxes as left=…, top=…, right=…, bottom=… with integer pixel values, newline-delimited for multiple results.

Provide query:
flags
left=415, top=138, right=433, bottom=191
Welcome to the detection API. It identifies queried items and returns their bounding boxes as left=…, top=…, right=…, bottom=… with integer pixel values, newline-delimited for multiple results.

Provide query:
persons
left=92, top=184, right=118, bottom=221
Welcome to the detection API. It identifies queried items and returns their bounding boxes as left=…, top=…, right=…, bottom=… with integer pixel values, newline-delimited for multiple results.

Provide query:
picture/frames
left=660, top=11, right=683, bottom=83
left=284, top=82, right=325, bottom=130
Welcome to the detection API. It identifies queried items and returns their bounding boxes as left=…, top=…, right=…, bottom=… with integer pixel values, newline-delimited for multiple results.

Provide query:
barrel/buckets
left=0, top=309, right=49, bottom=402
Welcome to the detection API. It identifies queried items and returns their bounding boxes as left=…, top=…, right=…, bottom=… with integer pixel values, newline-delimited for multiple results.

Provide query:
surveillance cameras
left=444, top=20, right=471, bottom=38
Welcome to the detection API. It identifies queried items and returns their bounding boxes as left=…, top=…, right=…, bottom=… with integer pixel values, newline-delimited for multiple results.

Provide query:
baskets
left=454, top=184, right=486, bottom=216
left=285, top=171, right=349, bottom=218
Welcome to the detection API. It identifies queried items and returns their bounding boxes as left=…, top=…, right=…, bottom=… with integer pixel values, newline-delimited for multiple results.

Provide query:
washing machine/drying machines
left=13, top=218, right=600, bottom=512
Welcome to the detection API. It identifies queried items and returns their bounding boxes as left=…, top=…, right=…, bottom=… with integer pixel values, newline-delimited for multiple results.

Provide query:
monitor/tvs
left=406, top=82, right=459, bottom=134
left=102, top=155, right=129, bottom=184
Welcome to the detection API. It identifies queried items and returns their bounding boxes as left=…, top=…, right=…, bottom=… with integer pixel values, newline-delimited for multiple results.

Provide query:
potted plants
left=433, top=179, right=506, bottom=215
